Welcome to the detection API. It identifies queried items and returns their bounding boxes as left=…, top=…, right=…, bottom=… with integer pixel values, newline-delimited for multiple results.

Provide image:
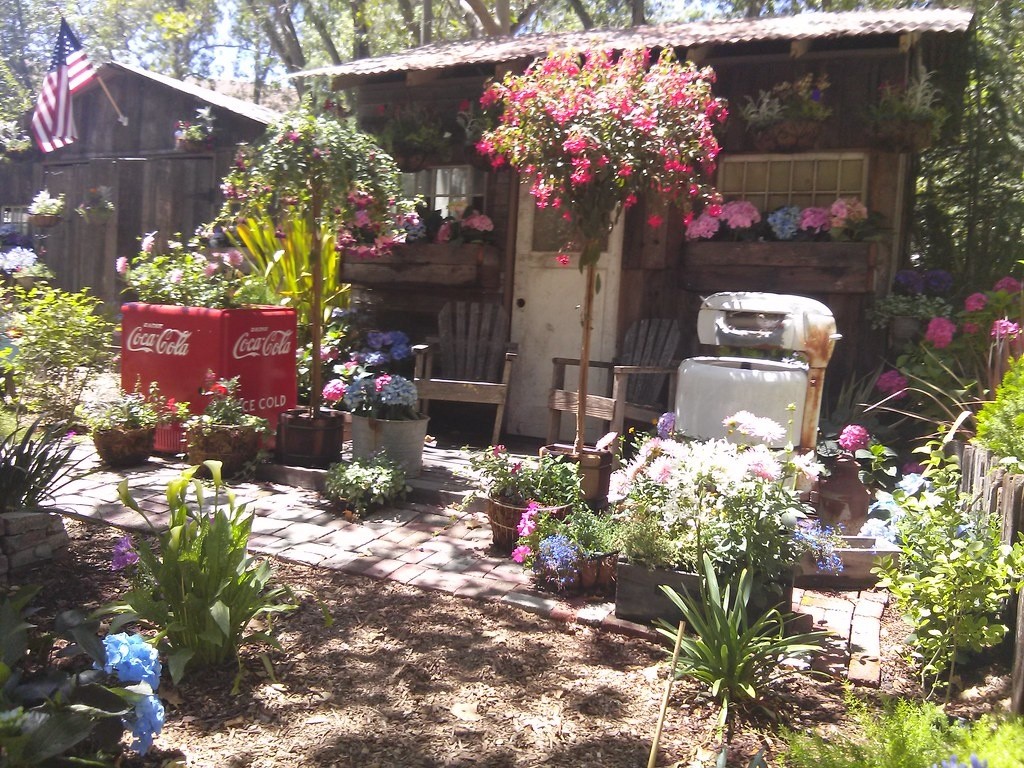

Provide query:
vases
left=682, top=240, right=888, bottom=294
left=84, top=215, right=108, bottom=224
left=181, top=139, right=207, bottom=153
left=391, top=150, right=437, bottom=174
left=465, top=146, right=509, bottom=172
left=93, top=430, right=156, bottom=465
left=28, top=215, right=60, bottom=228
left=615, top=559, right=797, bottom=633
left=342, top=241, right=498, bottom=287
left=353, top=413, right=430, bottom=479
left=205, top=247, right=258, bottom=274
left=188, top=423, right=257, bottom=479
left=277, top=409, right=346, bottom=469
left=539, top=443, right=613, bottom=500
left=752, top=119, right=826, bottom=150
left=867, top=121, right=935, bottom=153
left=488, top=491, right=569, bottom=551
left=18, top=276, right=54, bottom=290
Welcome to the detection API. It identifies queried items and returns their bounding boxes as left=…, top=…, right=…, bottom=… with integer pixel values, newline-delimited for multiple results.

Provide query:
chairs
left=549, top=317, right=681, bottom=469
left=411, top=300, right=518, bottom=448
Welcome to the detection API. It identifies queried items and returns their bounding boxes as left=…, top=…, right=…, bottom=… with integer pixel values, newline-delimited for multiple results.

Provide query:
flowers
left=0, top=34, right=937, bottom=619
